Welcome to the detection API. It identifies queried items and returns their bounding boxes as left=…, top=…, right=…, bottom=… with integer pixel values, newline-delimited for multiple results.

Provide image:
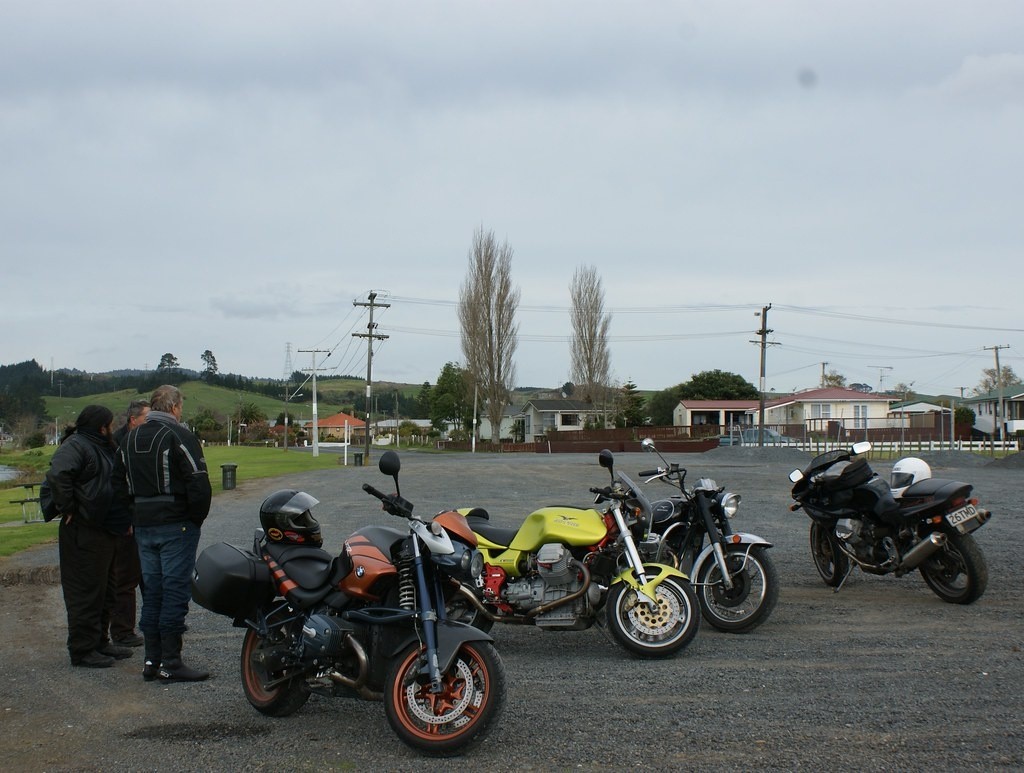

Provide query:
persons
left=111, top=401, right=189, bottom=648
left=117, top=384, right=212, bottom=684
left=40, top=404, right=134, bottom=668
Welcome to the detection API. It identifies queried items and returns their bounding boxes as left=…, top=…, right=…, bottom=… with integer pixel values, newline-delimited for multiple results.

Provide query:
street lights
left=283, top=393, right=304, bottom=451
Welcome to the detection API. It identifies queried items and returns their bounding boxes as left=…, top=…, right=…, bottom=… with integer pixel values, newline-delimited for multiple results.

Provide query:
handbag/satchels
left=840, top=458, right=873, bottom=489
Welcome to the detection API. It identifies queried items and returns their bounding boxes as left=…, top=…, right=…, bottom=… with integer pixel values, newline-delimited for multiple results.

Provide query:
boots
left=158, top=626, right=209, bottom=684
left=143, top=625, right=161, bottom=681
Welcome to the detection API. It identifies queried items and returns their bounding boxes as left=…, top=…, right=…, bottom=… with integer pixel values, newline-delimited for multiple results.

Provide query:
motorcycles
left=435, top=448, right=701, bottom=658
left=188, top=448, right=509, bottom=760
left=615, top=438, right=780, bottom=634
left=788, top=440, right=993, bottom=605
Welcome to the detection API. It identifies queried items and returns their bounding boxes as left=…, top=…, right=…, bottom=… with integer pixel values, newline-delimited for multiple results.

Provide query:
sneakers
left=96, top=645, right=133, bottom=660
left=113, top=634, right=144, bottom=646
left=71, top=651, right=115, bottom=667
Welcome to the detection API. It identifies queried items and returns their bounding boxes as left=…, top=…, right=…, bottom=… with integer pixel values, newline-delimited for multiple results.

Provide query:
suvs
left=718, top=427, right=799, bottom=448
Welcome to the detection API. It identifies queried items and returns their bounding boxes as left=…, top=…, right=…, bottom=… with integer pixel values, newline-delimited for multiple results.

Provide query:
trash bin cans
left=354, top=452, right=363, bottom=466
left=220, top=463, right=238, bottom=491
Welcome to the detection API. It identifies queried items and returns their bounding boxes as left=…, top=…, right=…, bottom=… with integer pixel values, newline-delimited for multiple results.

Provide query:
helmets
left=890, top=457, right=931, bottom=498
left=260, top=489, right=321, bottom=548
